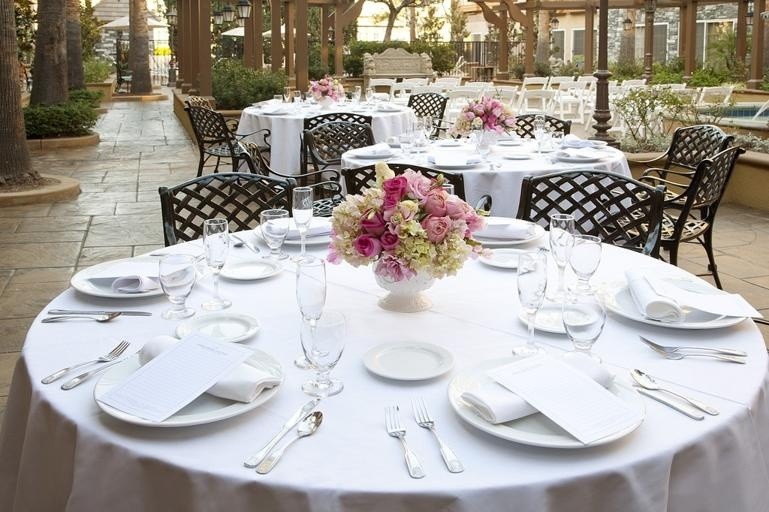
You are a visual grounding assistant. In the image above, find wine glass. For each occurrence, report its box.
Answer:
[299,309,348,396]
[154,251,197,321]
[275,82,374,112]
[517,212,609,359]
[288,186,318,265]
[401,115,545,161]
[203,217,233,309]
[258,209,292,263]
[292,257,327,370]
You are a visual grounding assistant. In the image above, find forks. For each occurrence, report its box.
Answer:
[380,402,427,481]
[636,336,750,370]
[41,340,131,386]
[39,310,125,326]
[410,395,465,476]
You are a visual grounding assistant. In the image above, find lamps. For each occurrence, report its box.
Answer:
[550,11,559,29]
[622,8,633,30]
[167,6,177,26]
[212,0,252,27]
[745,2,753,25]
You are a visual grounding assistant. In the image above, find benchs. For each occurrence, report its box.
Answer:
[362,47,434,93]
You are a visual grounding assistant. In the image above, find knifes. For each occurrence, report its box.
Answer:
[244,397,322,468]
[47,308,152,317]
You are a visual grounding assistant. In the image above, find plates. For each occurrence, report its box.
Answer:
[219,260,282,281]
[478,247,538,270]
[350,137,611,168]
[251,215,334,247]
[263,100,402,113]
[468,216,544,247]
[446,357,649,452]
[175,312,261,347]
[69,255,168,300]
[599,273,748,333]
[93,345,286,427]
[363,339,454,382]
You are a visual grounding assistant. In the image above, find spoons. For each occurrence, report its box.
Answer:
[258,410,329,478]
[628,368,722,420]
